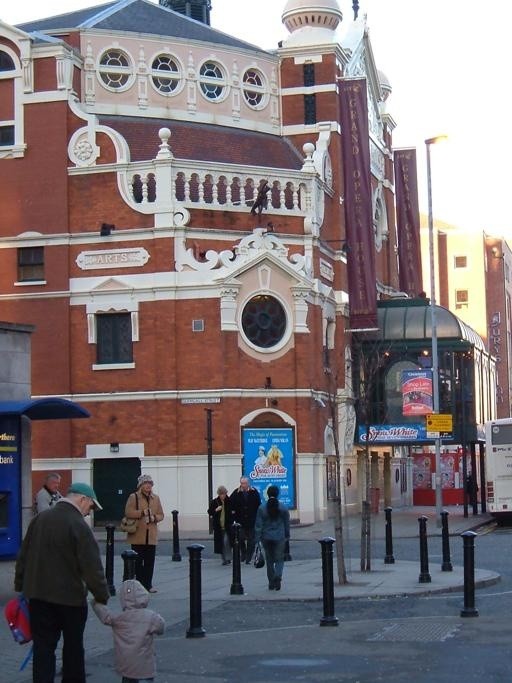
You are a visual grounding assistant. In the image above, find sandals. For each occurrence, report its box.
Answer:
[483,416,512,528]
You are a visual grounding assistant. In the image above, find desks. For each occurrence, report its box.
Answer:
[421,132,450,530]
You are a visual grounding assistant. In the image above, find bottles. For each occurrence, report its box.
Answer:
[137,474,153,488]
[68,483,102,511]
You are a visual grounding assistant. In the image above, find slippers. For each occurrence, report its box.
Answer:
[5,596,32,644]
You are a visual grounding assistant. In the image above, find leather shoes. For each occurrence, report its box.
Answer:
[144,515,155,523]
[143,508,151,516]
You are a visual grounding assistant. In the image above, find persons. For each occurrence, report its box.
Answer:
[228,475,260,563]
[90,577,167,682]
[13,481,111,681]
[207,485,232,565]
[254,444,268,466]
[124,472,164,593]
[254,485,292,590]
[34,470,63,515]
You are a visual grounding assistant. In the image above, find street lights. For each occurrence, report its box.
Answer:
[120,517,139,533]
[254,553,264,568]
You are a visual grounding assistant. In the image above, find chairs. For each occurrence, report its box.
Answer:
[223,560,229,564]
[149,588,156,593]
[241,555,251,563]
[284,555,291,560]
[269,576,281,590]
